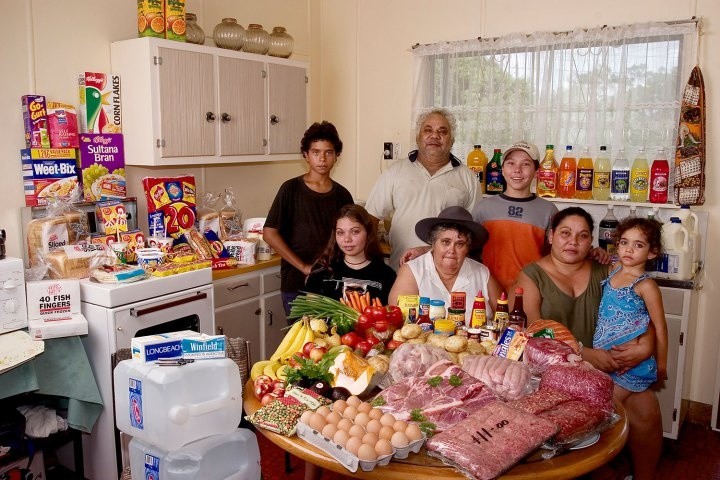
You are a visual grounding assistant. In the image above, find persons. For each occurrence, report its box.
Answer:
[365,107,483,277]
[389,205,506,326]
[400,142,612,295]
[263,120,354,333]
[303,204,398,337]
[516,205,670,480]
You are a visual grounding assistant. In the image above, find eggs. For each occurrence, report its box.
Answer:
[299,396,422,460]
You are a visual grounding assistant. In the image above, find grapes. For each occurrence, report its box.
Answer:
[82,162,126,189]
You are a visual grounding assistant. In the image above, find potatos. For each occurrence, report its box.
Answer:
[366,353,392,374]
[392,324,495,366]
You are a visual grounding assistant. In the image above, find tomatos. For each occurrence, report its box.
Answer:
[341,306,403,355]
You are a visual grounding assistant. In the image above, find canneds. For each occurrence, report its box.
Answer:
[429,300,456,338]
[245,229,272,260]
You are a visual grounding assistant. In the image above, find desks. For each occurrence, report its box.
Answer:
[245,378,629,480]
[0,334,104,480]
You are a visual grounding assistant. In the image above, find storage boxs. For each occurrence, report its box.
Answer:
[131,330,225,363]
[21,72,126,207]
[26,280,88,340]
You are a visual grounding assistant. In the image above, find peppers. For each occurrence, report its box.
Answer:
[384,305,402,327]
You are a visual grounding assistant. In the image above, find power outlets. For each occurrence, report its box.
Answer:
[384,143,402,160]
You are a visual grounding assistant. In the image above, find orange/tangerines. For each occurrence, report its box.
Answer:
[138,13,186,35]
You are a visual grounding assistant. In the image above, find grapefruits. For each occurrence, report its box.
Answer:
[326,352,378,396]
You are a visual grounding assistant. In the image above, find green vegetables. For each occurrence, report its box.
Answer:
[371,376,462,438]
[282,352,337,383]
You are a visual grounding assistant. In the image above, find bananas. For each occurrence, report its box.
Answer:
[270,314,314,363]
[251,360,289,380]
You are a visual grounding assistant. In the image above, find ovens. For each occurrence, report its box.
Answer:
[80,268,217,480]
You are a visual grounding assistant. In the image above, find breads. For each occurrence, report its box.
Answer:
[183,209,259,258]
[25,212,107,281]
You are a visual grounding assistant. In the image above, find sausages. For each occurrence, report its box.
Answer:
[461,356,528,400]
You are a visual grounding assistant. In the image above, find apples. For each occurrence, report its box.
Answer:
[253,376,287,406]
[289,343,328,369]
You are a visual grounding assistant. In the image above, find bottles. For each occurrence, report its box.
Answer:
[577,148,593,200]
[493,292,510,334]
[434,319,455,336]
[485,148,507,195]
[630,148,650,203]
[111,242,127,264]
[184,13,293,58]
[467,145,488,194]
[558,146,576,199]
[609,146,631,201]
[536,144,560,198]
[509,288,528,333]
[468,328,481,343]
[593,146,611,200]
[470,290,487,329]
[429,300,446,322]
[414,297,435,334]
[447,307,466,328]
[598,204,702,280]
[649,146,670,203]
[247,230,271,261]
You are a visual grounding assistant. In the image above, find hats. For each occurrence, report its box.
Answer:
[415,206,490,256]
[501,142,539,171]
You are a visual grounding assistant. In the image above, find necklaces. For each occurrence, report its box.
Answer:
[343,256,368,265]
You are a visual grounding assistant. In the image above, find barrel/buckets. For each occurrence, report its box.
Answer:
[112,354,244,452]
[128,428,261,480]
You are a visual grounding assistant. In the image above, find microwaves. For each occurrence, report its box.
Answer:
[0,256,28,335]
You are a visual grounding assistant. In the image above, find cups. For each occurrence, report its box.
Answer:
[136,248,164,269]
[498,315,525,339]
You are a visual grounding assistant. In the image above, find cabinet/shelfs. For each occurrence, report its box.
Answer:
[214,265,287,367]
[110,37,310,166]
[656,286,699,439]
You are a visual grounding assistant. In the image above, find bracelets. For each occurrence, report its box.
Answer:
[578,342,583,356]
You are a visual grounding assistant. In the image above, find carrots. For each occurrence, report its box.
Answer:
[339,290,382,314]
